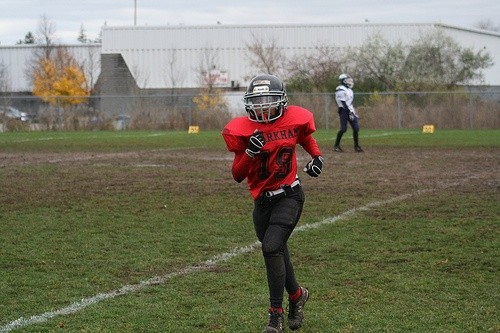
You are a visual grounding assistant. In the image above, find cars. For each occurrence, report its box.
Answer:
[66,106,134,132]
[0,105,29,133]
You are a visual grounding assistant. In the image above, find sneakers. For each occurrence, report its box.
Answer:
[262,306,284,333]
[285,287,309,331]
[333,145,344,153]
[354,145,363,152]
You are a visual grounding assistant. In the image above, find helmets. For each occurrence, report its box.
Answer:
[243,75,288,122]
[338,74,354,88]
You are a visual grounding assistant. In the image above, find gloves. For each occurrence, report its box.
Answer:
[306,155,323,177]
[245,131,265,158]
[348,112,355,122]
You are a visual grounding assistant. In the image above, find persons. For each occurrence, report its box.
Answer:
[222,75,324,333]
[332,73,365,152]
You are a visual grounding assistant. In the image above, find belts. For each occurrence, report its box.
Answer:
[262,174,299,197]
